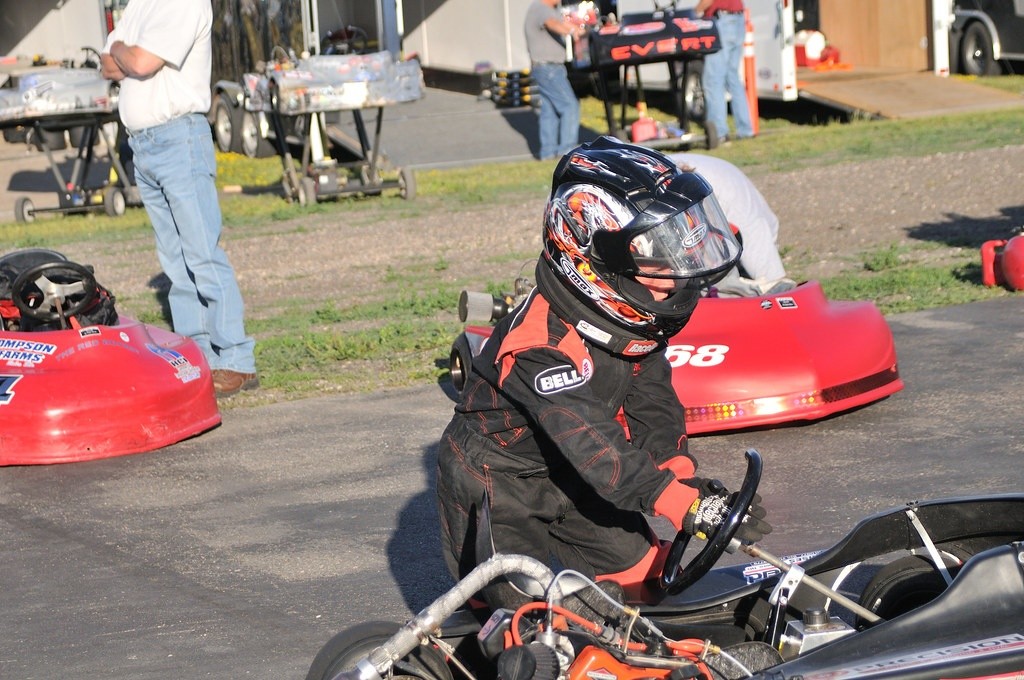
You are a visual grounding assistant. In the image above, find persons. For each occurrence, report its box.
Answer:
[524,0,585,160]
[435,135,772,632]
[693,0,756,143]
[101,0,261,396]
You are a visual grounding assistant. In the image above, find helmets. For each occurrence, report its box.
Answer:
[535,135,741,357]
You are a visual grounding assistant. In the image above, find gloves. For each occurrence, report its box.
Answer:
[677,476,772,541]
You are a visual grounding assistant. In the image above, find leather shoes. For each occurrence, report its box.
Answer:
[211,369,258,398]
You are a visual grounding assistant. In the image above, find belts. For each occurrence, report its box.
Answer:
[716,8,744,16]
